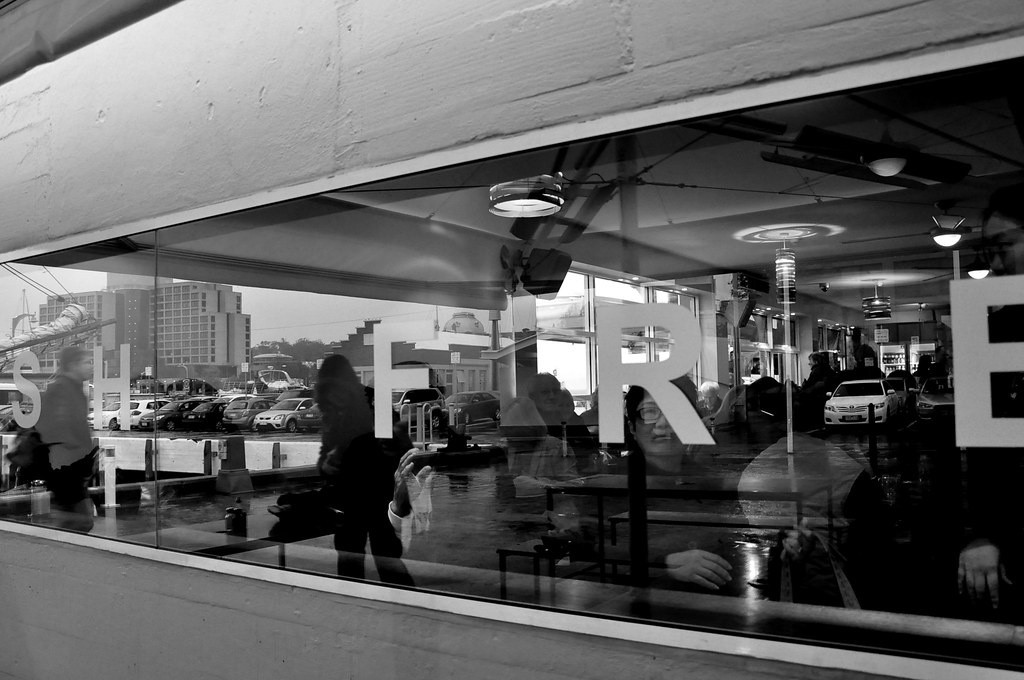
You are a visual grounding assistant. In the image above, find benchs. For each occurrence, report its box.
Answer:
[495,536,703,603]
[607,511,850,590]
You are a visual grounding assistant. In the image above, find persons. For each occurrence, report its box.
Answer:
[800,353,835,390]
[624,376,733,590]
[933,429,1024,624]
[37,346,97,530]
[389,389,516,573]
[316,355,417,586]
[501,374,597,515]
[760,472,891,610]
[699,380,723,416]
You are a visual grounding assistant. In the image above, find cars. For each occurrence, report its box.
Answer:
[824,378,901,432]
[0,369,322,434]
[912,376,955,424]
[442,390,501,426]
[881,377,910,414]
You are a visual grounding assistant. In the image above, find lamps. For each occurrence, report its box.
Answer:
[931,232,962,248]
[861,283,893,320]
[489,175,563,219]
[967,269,990,279]
[864,158,908,177]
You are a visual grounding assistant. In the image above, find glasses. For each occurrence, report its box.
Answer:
[635,407,663,419]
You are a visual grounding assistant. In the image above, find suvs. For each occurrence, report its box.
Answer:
[372,387,446,430]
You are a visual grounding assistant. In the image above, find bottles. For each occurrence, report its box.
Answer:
[224,496,248,537]
[30,480,46,515]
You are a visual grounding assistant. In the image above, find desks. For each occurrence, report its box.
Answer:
[540,473,832,603]
